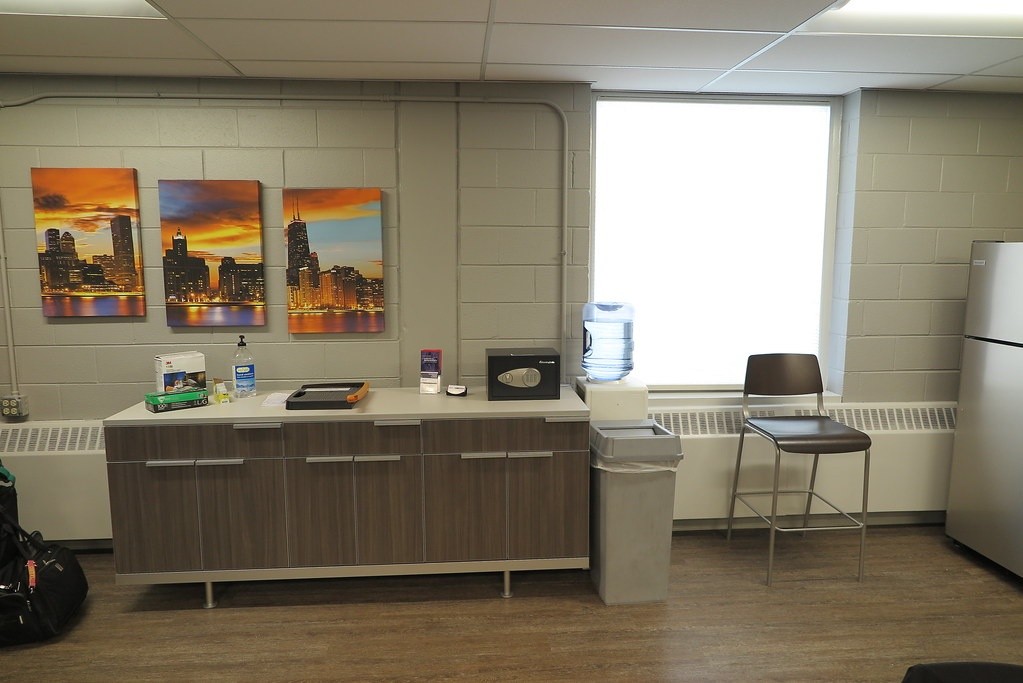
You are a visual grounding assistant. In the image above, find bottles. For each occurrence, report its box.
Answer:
[581,301,635,381]
[231,336,256,398]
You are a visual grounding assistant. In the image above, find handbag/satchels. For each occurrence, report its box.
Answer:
[0,510,89,646]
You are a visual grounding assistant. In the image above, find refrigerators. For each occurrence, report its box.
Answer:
[944,239,1022,584]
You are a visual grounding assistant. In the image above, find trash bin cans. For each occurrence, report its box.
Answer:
[589,420,684,606]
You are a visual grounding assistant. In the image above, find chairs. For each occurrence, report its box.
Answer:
[724,353,872,587]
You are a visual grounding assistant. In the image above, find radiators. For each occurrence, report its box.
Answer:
[0,420,114,550]
[648,401,959,532]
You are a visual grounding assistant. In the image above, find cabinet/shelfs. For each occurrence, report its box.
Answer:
[102,383,591,609]
